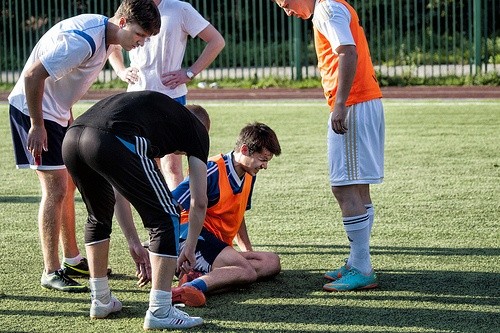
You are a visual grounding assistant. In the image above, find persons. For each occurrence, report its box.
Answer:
[107,0,225,247]
[170,119,281,306]
[62,90,210,330]
[8,0,160,292]
[275,0,385,292]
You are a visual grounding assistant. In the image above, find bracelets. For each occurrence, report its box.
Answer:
[185,68,196,79]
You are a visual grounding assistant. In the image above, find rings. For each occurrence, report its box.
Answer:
[38,145,42,146]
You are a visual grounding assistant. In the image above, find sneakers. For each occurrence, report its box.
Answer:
[90,290,123,318]
[143,305,204,330]
[177,269,202,287]
[324,262,352,281]
[323,265,378,291]
[171,282,206,307]
[41,268,88,292]
[61,258,112,278]
